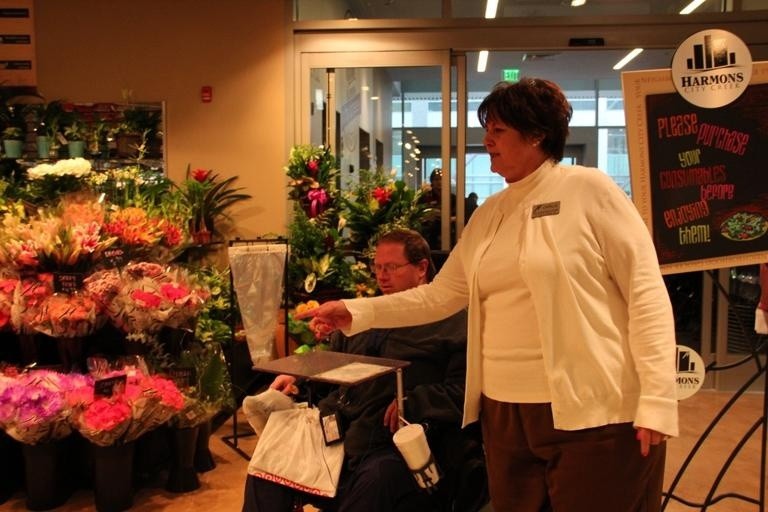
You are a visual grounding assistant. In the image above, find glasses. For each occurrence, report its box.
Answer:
[369,258,421,275]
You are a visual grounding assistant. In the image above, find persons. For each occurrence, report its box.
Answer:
[292,76,680,512]
[465,192,479,225]
[416,167,456,250]
[239,226,489,511]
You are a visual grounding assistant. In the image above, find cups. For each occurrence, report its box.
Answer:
[392,424,440,489]
